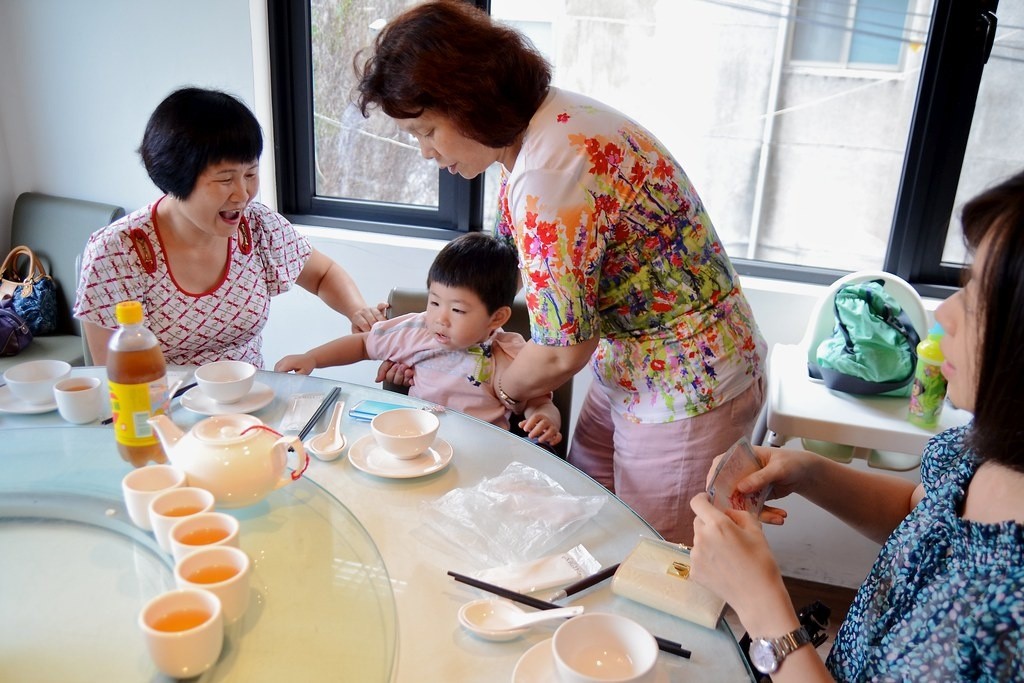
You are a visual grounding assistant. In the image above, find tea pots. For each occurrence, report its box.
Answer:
[147,415,308,509]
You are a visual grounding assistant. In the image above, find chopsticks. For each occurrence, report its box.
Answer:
[101,382,197,425]
[447,571,691,659]
[287,387,341,452]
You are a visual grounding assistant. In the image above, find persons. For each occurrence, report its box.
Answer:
[689,170,1024,683]
[277,232,562,445]
[73,89,390,371]
[360,0,768,547]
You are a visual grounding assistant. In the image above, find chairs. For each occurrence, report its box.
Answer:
[0,190,126,376]
[383,286,571,466]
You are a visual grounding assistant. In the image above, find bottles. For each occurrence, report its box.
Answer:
[107,301,170,468]
[907,322,947,430]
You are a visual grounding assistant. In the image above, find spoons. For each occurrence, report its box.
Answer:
[462,601,584,631]
[312,400,345,451]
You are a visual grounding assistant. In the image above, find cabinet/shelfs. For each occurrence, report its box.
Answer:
[749,345,976,588]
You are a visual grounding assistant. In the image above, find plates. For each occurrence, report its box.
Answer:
[0,371,57,414]
[180,382,275,415]
[511,637,670,683]
[348,434,453,478]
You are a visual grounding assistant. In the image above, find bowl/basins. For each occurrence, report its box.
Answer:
[551,613,659,683]
[3,359,72,404]
[458,602,530,641]
[303,432,346,461]
[371,409,439,460]
[195,361,256,404]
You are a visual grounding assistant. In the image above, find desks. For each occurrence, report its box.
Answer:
[0,366,757,683]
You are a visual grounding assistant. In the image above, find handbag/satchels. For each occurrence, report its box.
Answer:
[0,246,58,334]
[0,305,33,357]
[819,282,920,397]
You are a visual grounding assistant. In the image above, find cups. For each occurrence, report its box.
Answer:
[122,465,187,531]
[173,546,250,625]
[148,488,216,554]
[54,376,101,424]
[170,512,239,562]
[138,589,223,678]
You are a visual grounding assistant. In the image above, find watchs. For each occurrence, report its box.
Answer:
[498,376,517,406]
[749,626,811,674]
